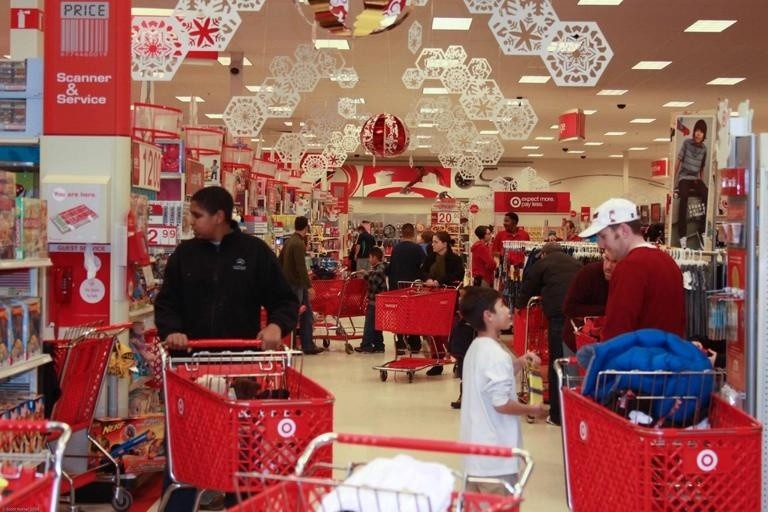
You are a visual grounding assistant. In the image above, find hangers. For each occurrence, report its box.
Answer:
[501,240,704,266]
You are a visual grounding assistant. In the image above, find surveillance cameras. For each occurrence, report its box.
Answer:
[562,148,569,153]
[617,103,626,110]
[581,156,586,159]
[229,62,241,75]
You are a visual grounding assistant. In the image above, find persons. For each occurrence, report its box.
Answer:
[458,287,550,511]
[277,217,325,356]
[560,245,618,388]
[352,224,466,375]
[676,120,708,250]
[493,213,530,269]
[576,197,686,340]
[470,226,497,287]
[513,243,585,426]
[545,220,582,242]
[156,185,300,511]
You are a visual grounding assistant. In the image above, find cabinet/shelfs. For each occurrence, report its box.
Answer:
[255,213,469,281]
[0,257,54,469]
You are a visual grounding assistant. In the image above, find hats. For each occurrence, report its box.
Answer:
[577,198,640,238]
[535,242,562,258]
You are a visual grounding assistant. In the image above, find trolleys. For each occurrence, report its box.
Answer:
[375,278,464,383]
[513,295,548,405]
[43,318,136,511]
[156,337,335,511]
[569,315,601,385]
[1,418,73,511]
[219,431,534,511]
[308,264,371,355]
[553,350,763,510]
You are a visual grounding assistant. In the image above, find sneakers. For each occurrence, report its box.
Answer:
[427,366,443,376]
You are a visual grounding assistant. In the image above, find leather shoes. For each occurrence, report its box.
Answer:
[311,346,424,355]
